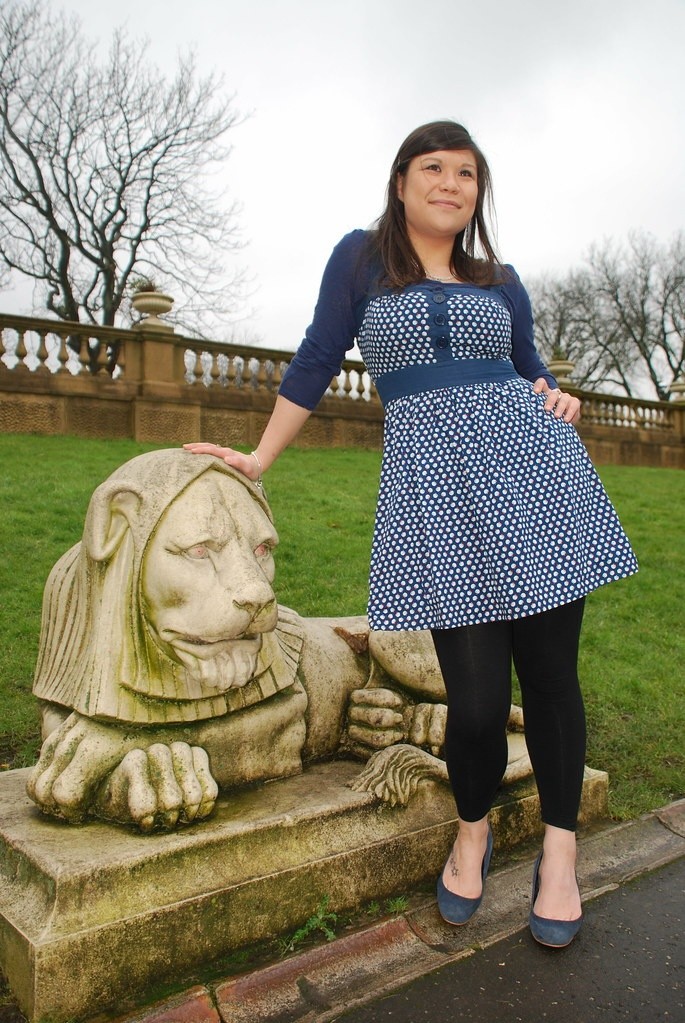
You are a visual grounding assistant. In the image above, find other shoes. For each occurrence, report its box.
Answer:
[528,848,584,947]
[437,824,493,926]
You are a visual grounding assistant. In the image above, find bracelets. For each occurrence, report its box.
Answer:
[251,452,263,489]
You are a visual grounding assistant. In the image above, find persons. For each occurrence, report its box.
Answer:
[183,120,639,947]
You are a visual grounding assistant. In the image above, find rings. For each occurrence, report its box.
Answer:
[216,444,222,449]
[552,388,562,399]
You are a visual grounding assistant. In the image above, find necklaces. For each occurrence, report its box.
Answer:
[415,259,457,279]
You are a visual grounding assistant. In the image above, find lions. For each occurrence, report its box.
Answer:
[25,448,534,835]
